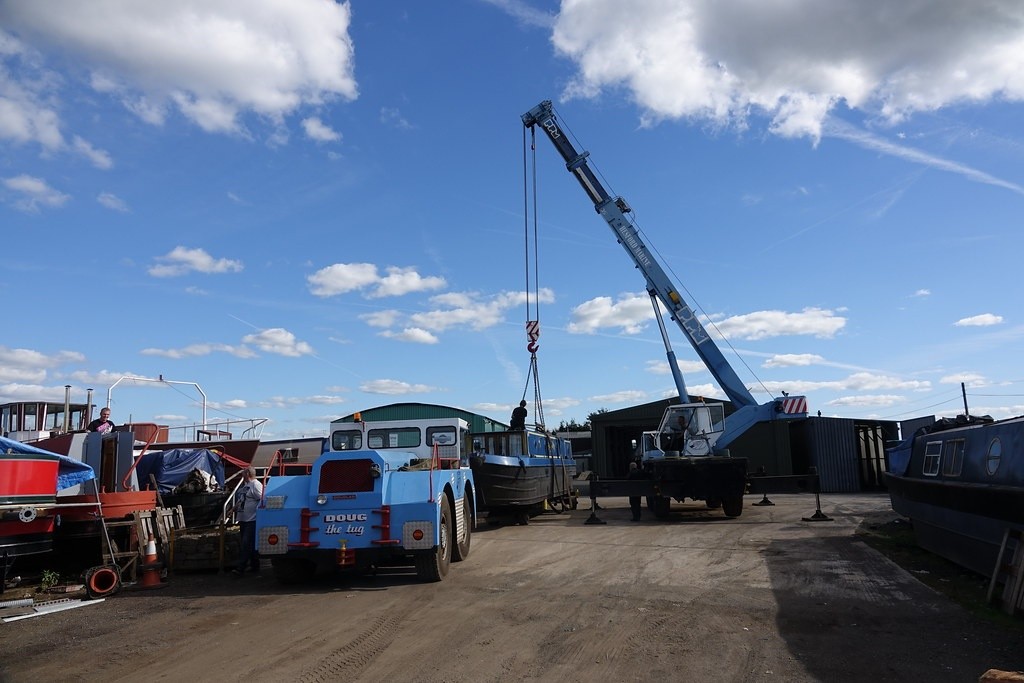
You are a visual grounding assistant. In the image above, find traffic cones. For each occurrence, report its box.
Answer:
[130,533,170,590]
[85,563,123,601]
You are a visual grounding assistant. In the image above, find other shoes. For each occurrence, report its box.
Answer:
[631,518,640,521]
[233,567,244,574]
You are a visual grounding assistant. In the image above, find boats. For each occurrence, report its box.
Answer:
[0,377,206,575]
[470,429,577,506]
[132,446,231,527]
[881,415,1024,585]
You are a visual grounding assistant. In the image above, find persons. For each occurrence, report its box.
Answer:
[227,466,264,575]
[510,400,527,430]
[86,407,116,435]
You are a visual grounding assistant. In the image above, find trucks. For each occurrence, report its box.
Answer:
[254,412,479,592]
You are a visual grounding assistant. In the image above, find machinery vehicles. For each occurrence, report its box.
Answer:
[519,98,836,526]
